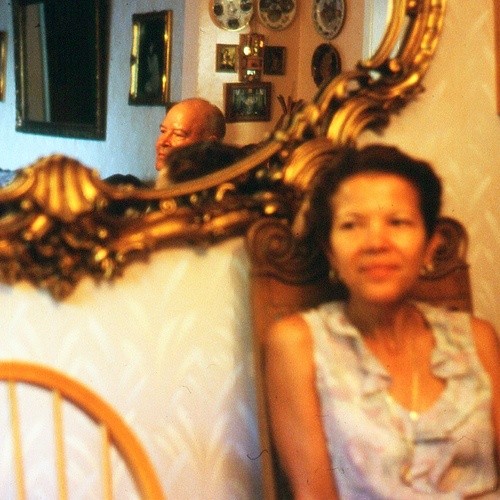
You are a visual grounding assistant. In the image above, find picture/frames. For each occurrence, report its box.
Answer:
[128,9,172,107]
[263,45,287,75]
[223,82,272,122]
[215,44,242,73]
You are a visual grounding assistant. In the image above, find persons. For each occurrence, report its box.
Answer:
[151,98,225,172]
[153,140,258,191]
[264,143,499,500]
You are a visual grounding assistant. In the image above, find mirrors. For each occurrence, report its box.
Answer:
[0,1,449,302]
[10,0,112,141]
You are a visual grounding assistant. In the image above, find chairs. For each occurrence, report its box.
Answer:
[2,360,164,498]
[242,185,475,499]
